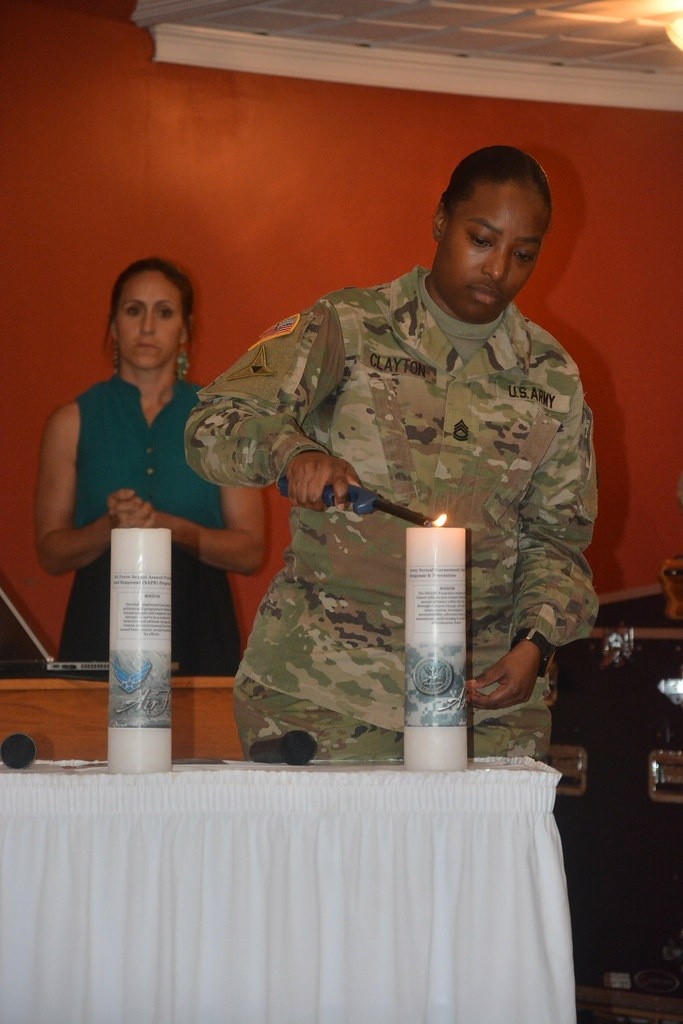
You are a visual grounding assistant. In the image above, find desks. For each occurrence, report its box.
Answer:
[0,675,240,766]
[3,752,579,1022]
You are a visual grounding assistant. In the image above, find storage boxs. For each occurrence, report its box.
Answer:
[543,580,683,994]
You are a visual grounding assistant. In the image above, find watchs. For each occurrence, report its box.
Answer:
[509,625,559,679]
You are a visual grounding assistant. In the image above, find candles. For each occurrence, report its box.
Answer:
[403,513,473,770]
[103,525,176,773]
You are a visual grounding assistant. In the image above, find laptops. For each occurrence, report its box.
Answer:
[1,589,180,674]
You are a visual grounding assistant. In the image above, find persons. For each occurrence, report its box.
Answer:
[30,257,269,677]
[183,142,598,761]
